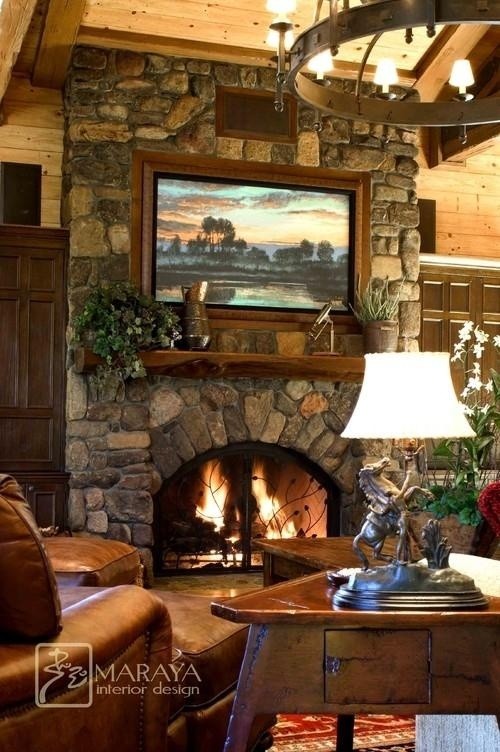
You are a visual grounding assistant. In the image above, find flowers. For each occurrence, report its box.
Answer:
[421,320,500,527]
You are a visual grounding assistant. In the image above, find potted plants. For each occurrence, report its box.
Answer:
[71,276,183,403]
[348,268,414,353]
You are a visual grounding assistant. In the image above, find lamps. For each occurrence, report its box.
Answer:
[334,350,487,610]
[265,0,500,141]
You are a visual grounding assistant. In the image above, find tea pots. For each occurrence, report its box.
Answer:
[180,280,214,352]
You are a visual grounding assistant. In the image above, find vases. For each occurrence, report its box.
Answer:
[368,505,496,563]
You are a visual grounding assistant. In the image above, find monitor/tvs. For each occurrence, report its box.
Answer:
[141,161,363,324]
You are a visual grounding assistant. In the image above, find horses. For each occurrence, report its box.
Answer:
[352,456,431,571]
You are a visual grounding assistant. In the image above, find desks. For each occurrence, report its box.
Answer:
[210,532,498,752]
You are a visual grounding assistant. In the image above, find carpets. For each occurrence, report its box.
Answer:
[265,712,417,752]
